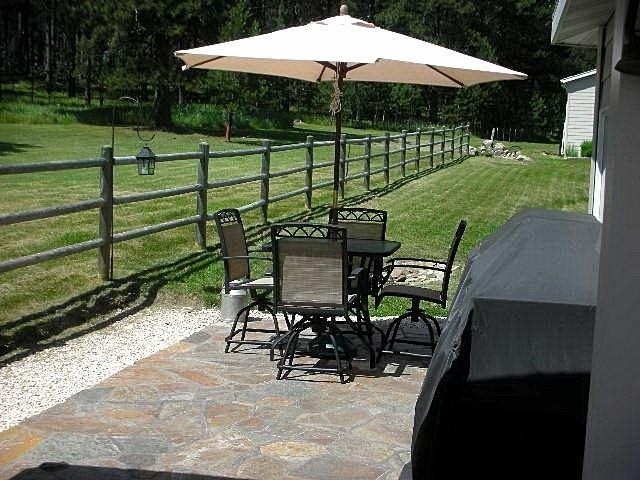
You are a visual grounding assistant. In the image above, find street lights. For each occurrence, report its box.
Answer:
[107,94,157,279]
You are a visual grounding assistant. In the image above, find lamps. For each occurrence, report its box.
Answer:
[137,141,156,174]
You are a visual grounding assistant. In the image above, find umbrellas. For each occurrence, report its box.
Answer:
[173,4,528,241]
